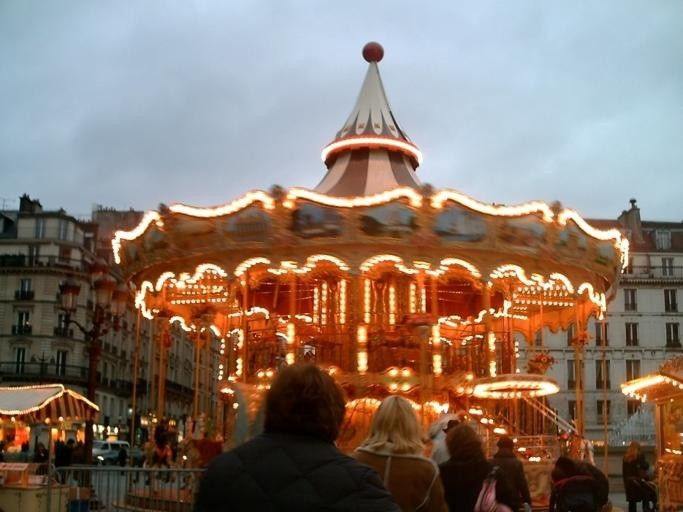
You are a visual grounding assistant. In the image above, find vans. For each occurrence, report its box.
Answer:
[92,440,131,464]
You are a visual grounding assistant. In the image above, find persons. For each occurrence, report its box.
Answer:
[118,444,127,475]
[431,419,532,512]
[192,363,393,512]
[622,440,656,511]
[146,432,172,468]
[63,437,77,457]
[350,395,448,512]
[546,456,609,512]
[33,442,48,473]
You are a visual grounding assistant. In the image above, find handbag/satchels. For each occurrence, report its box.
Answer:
[474,478,512,512]
